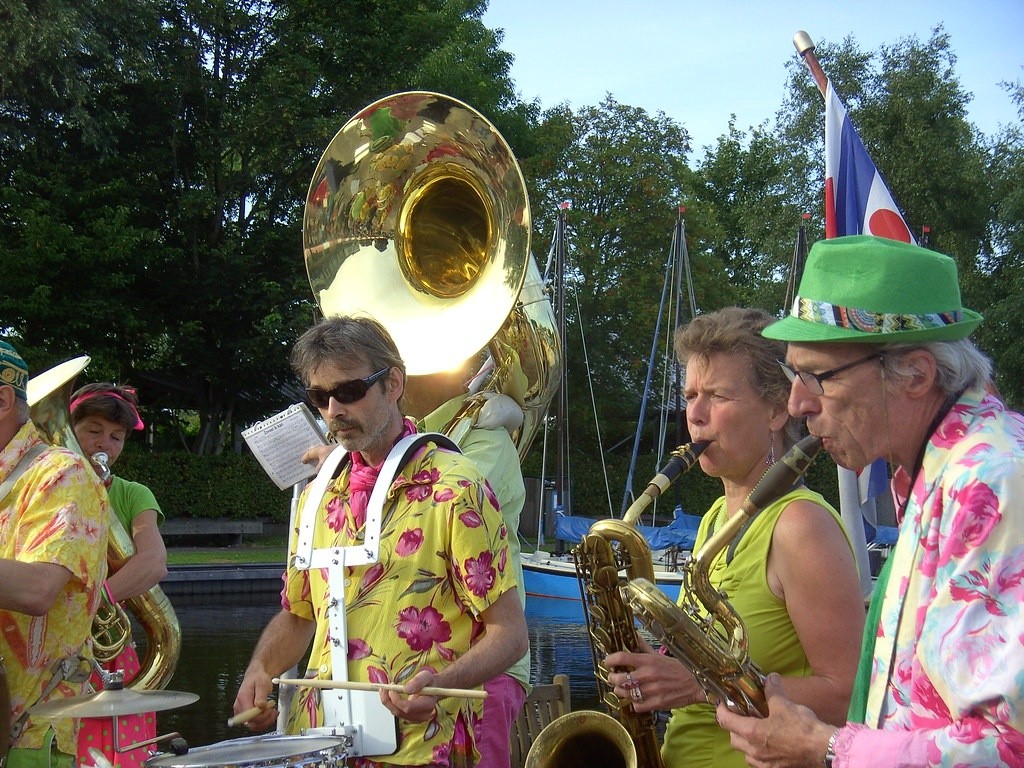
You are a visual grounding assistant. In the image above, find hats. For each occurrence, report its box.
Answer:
[0,340,29,401]
[760,234,986,343]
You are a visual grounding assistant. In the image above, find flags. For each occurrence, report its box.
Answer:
[825,78,917,601]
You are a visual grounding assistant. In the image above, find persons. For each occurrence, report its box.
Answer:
[312,93,523,235]
[234,315,529,768]
[68,381,168,768]
[0,337,110,768]
[604,236,1024,768]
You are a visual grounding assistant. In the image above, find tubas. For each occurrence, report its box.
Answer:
[23,354,183,692]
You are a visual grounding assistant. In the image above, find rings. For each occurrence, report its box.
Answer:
[629,690,642,701]
[620,672,642,688]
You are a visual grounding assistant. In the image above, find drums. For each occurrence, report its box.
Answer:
[143,733,351,768]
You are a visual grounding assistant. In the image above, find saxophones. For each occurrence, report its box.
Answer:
[616,433,826,719]
[521,438,717,768]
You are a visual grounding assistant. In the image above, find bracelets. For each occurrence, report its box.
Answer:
[98,581,114,611]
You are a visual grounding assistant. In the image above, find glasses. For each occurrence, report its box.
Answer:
[775,348,882,396]
[305,367,389,408]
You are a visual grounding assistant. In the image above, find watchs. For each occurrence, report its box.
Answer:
[825,728,839,768]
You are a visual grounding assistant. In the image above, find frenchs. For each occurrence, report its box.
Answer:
[300,87,565,468]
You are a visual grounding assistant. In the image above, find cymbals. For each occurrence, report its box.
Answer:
[24,686,200,721]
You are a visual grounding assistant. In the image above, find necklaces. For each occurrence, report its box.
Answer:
[715,461,779,530]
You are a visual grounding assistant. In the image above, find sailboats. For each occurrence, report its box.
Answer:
[516,200,901,615]
